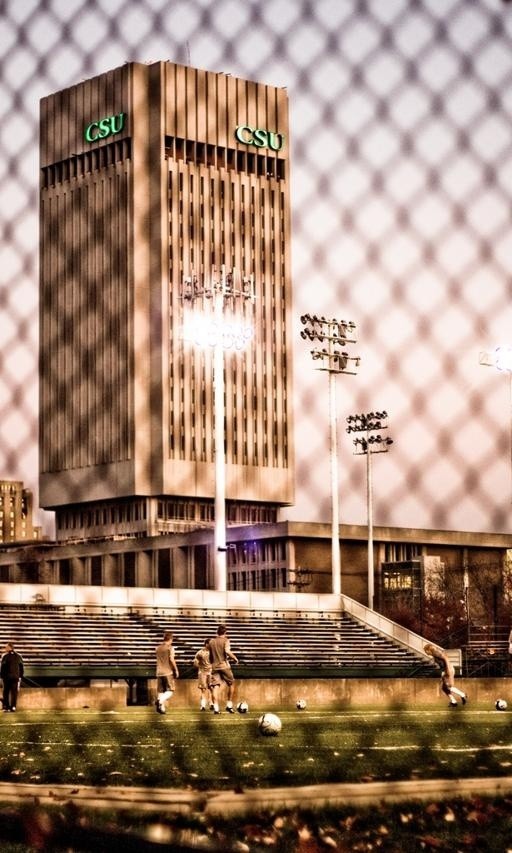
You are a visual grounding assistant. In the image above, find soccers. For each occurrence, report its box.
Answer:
[495,698,507,711]
[236,702,248,713]
[296,700,306,710]
[258,713,281,736]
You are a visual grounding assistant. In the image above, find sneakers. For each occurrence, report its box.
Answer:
[448,691,467,707]
[200,705,234,715]
[156,698,165,713]
[4,706,16,712]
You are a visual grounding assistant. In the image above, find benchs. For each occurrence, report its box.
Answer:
[0,607,437,670]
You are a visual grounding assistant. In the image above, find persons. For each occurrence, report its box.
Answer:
[154,633,180,714]
[192,638,214,711]
[208,625,239,714]
[0,643,26,713]
[423,644,468,708]
